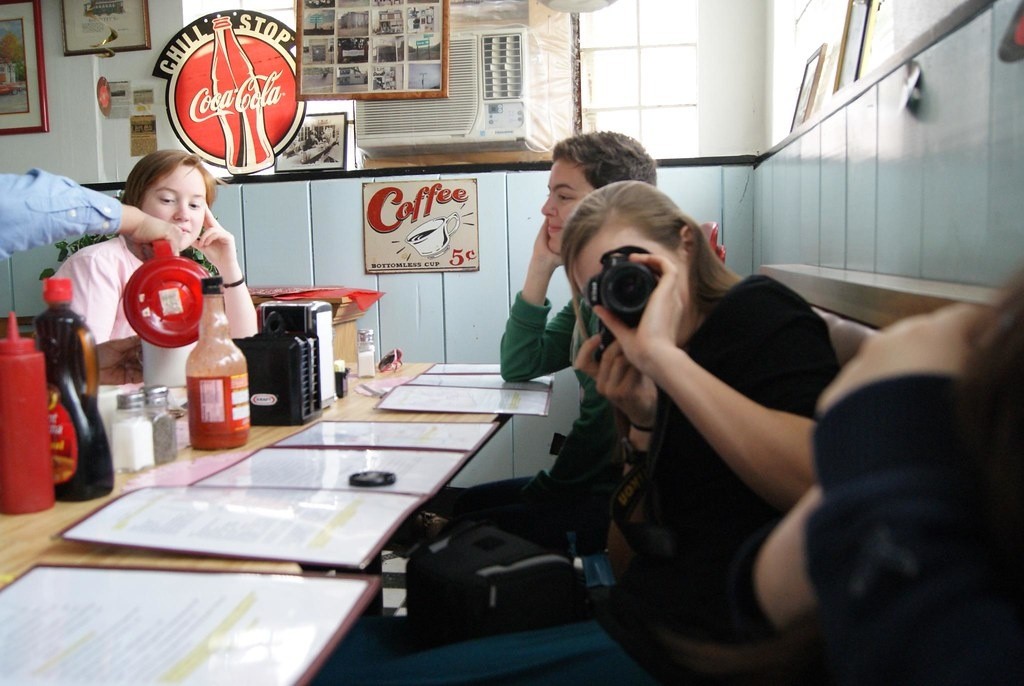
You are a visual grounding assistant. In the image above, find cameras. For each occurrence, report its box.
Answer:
[586,255,660,361]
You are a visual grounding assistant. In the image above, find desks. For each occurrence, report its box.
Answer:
[0,362,513,686]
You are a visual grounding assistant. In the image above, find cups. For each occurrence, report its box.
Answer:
[139,337,199,386]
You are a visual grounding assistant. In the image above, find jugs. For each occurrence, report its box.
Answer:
[123,239,213,349]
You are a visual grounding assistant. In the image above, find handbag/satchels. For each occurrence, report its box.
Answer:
[405,521,584,643]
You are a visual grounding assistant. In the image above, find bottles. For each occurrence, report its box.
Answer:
[141,386,178,463]
[26,279,115,500]
[110,391,156,472]
[356,329,376,378]
[0,308,56,515]
[182,275,249,450]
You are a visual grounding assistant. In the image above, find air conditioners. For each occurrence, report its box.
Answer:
[351,24,533,157]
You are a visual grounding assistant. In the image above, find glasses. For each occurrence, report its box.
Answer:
[379,348,403,372]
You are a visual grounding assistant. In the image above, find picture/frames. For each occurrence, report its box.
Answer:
[789,44,828,133]
[295,0,450,102]
[60,0,152,56]
[0,0,49,135]
[274,111,348,175]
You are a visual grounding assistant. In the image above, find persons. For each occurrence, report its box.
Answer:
[310,130,1024,686]
[1,167,181,388]
[49,149,258,345]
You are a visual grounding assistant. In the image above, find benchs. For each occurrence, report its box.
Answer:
[751,262,1004,373]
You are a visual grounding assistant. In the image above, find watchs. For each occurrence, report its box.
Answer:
[620,437,648,466]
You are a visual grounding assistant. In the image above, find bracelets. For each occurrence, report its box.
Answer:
[223,277,244,288]
[632,422,654,432]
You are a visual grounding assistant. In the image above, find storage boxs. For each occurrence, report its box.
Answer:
[249,296,368,366]
